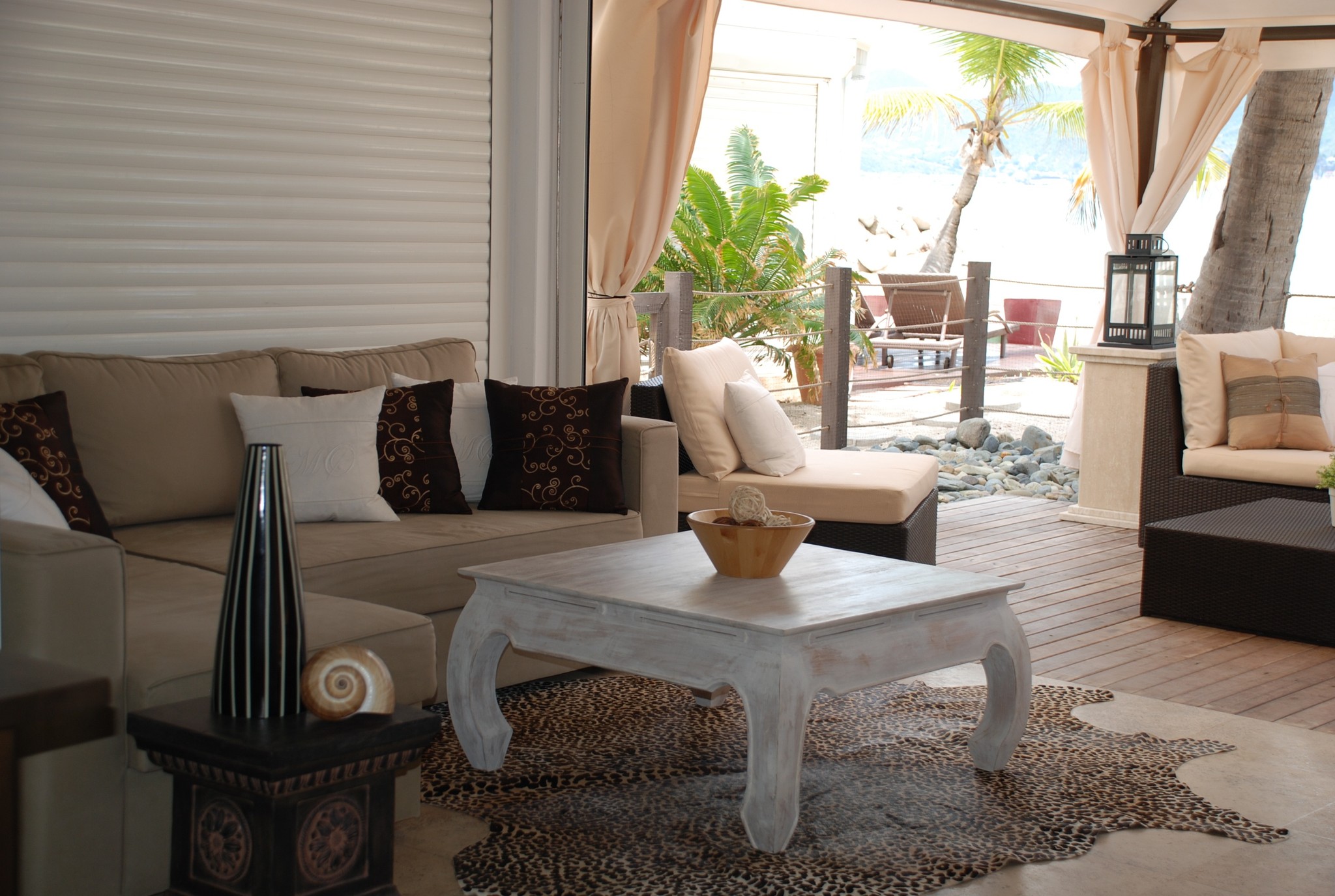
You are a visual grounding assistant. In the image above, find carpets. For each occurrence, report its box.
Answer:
[411,668,1291,896]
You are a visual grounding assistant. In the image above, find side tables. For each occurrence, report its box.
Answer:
[1056,343,1180,530]
[124,696,444,896]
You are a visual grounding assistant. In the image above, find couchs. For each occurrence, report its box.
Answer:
[0,336,678,896]
[629,337,938,569]
[1136,325,1335,548]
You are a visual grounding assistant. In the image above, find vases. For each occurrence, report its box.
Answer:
[205,443,310,725]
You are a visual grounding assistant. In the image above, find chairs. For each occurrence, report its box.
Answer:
[862,273,1020,371]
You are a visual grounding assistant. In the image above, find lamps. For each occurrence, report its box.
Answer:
[1097,233,1179,351]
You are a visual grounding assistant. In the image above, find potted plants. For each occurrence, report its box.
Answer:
[724,125,880,409]
[1314,450,1335,528]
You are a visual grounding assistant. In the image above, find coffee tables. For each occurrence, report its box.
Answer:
[1136,496,1335,650]
[443,526,1035,858]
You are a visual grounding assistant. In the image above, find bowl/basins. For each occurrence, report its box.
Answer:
[686,509,815,579]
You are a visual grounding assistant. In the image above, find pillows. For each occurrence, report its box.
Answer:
[229,384,404,529]
[301,378,473,517]
[661,337,762,482]
[476,377,631,517]
[1219,351,1335,452]
[1275,328,1335,367]
[389,372,520,503]
[0,390,123,545]
[722,367,808,479]
[0,448,72,530]
[1317,360,1335,448]
[1175,326,1283,450]
